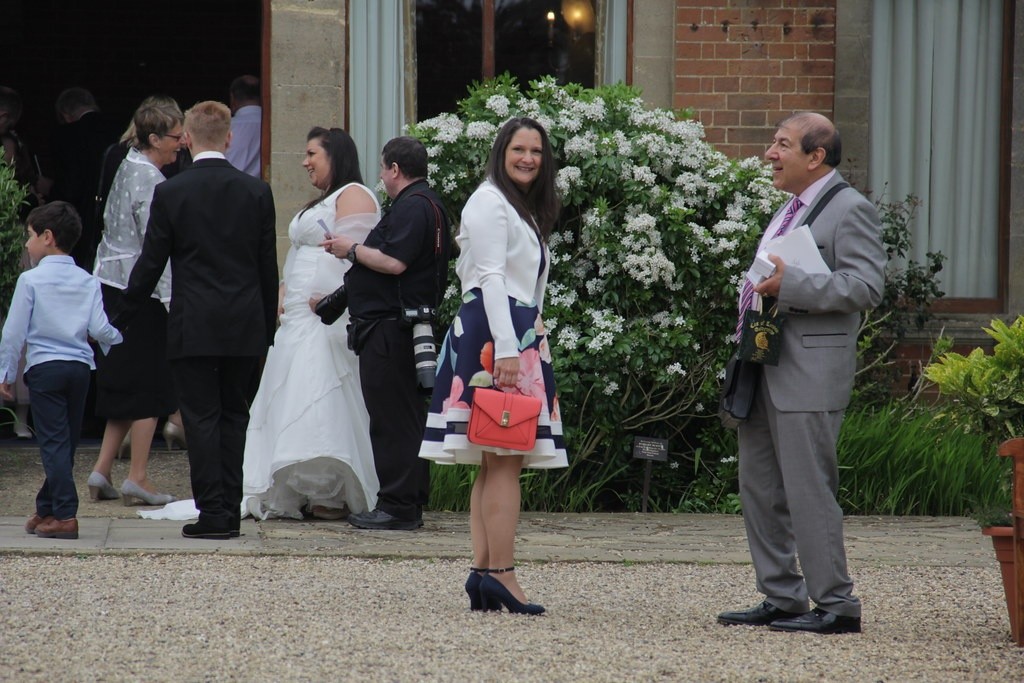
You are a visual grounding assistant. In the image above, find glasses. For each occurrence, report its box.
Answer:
[164,134,183,142]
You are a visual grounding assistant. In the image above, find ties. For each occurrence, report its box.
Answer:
[735,199,804,344]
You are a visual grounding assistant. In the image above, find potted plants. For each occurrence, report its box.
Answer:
[969,505,1017,642]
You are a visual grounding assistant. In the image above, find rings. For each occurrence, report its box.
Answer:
[761,292,769,297]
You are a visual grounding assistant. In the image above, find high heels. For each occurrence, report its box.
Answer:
[120,478,177,506]
[117,432,131,459]
[87,472,121,500]
[163,421,187,451]
[465,566,545,614]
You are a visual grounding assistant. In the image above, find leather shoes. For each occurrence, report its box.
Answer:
[25,513,78,539]
[347,509,424,530]
[717,600,810,627]
[769,606,861,635]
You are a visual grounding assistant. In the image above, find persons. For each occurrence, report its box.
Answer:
[315,135,452,531]
[88,94,187,506]
[0,198,122,539]
[0,75,260,193]
[119,100,279,538]
[713,109,889,636]
[137,125,382,521]
[418,118,569,615]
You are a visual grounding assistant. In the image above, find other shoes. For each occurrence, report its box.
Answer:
[182,518,241,540]
[314,504,350,519]
[13,428,32,438]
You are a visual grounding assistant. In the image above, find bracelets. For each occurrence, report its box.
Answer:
[346,243,358,263]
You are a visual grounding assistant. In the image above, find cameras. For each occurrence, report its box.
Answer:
[314,284,349,325]
[403,304,437,388]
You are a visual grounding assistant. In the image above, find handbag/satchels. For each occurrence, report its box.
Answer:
[86,246,96,273]
[718,353,761,430]
[740,291,787,368]
[468,383,542,452]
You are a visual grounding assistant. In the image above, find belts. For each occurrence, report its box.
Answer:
[356,315,397,322]
